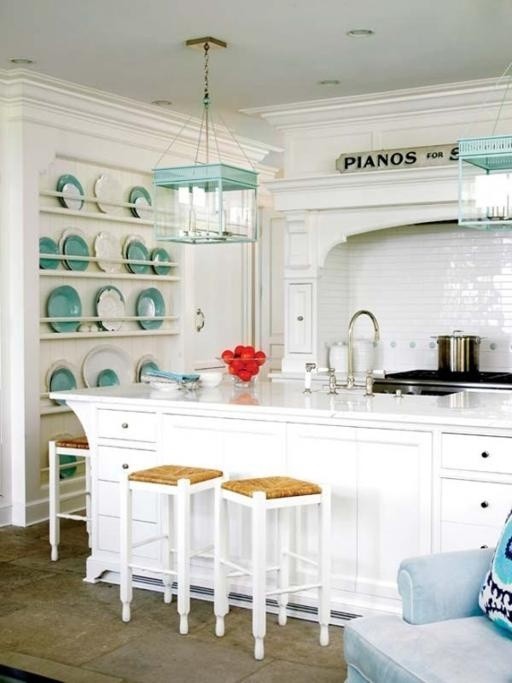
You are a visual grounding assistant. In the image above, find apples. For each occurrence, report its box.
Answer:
[222,345,266,381]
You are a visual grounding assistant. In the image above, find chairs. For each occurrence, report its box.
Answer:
[342,547,510,681]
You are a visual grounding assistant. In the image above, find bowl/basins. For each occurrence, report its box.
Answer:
[216,357,266,386]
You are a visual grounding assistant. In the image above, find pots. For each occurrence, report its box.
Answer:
[437,329,487,374]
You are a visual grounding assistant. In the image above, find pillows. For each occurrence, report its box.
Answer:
[477,506,512,635]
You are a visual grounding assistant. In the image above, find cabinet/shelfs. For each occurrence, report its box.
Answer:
[9,143,190,526]
[180,228,255,371]
[281,276,320,376]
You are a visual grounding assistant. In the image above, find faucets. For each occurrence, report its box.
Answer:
[347,310,380,390]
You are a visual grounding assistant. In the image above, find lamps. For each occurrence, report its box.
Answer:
[456,61,511,232]
[151,34,259,245]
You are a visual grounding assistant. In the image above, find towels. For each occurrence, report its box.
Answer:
[140,374,176,383]
[147,368,201,383]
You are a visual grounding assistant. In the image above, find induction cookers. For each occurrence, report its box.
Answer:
[373,369,512,395]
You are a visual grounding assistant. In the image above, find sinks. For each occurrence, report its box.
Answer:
[370,380,463,398]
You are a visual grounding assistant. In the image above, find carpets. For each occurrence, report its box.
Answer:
[1,663,66,683]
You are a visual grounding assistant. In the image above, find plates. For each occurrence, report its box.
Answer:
[94,285,127,331]
[93,173,121,214]
[96,288,125,330]
[137,356,160,381]
[139,361,158,382]
[59,454,77,477]
[81,345,133,386]
[59,228,91,270]
[129,186,151,218]
[123,233,149,273]
[47,363,76,390]
[151,247,172,274]
[134,195,150,216]
[126,241,149,273]
[94,232,124,271]
[63,235,90,270]
[136,286,165,328]
[49,370,76,404]
[62,182,81,209]
[38,236,59,268]
[97,368,119,386]
[57,172,84,209]
[46,285,82,331]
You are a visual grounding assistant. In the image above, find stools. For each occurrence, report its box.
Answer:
[223,476,331,662]
[121,465,228,638]
